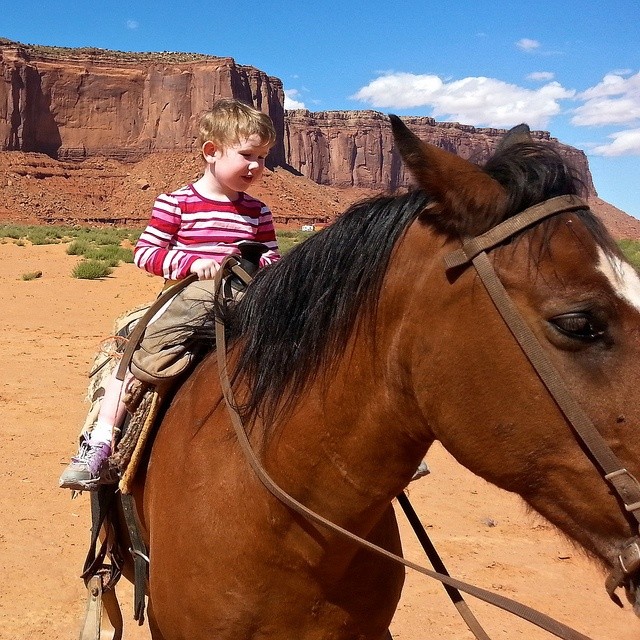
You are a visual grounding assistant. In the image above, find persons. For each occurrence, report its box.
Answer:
[60,97,282,495]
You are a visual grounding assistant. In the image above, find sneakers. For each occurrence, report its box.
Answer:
[59,441,109,489]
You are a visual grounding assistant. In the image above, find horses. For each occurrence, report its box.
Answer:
[90,112,639,639]
